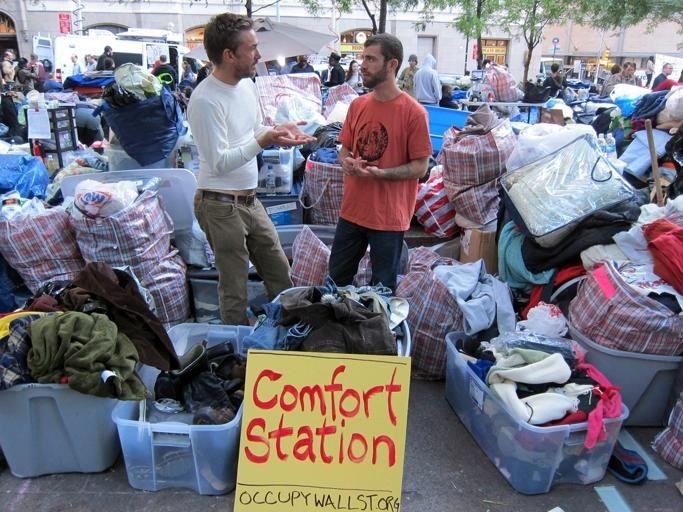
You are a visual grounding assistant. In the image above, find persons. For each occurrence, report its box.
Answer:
[152,56,215,112]
[0,49,47,94]
[185,11,318,325]
[537,56,674,97]
[324,32,434,299]
[70,46,116,72]
[287,49,460,110]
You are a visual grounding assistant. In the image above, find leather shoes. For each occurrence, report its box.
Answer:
[154,339,246,423]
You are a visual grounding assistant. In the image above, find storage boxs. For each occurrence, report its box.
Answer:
[0,76,682,492]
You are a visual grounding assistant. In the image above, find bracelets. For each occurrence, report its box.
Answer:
[340,151,354,167]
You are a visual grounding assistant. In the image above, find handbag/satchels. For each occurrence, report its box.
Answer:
[652,392,683,468]
[289,225,461,383]
[567,257,683,356]
[482,64,525,102]
[414,117,636,246]
[92,75,187,165]
[254,72,360,226]
[0,179,193,330]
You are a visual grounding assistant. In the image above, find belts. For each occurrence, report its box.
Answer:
[204,190,257,206]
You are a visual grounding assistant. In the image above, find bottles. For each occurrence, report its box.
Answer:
[266,164,276,195]
[47,155,55,174]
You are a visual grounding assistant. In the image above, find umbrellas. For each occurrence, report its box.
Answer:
[183,15,338,65]
